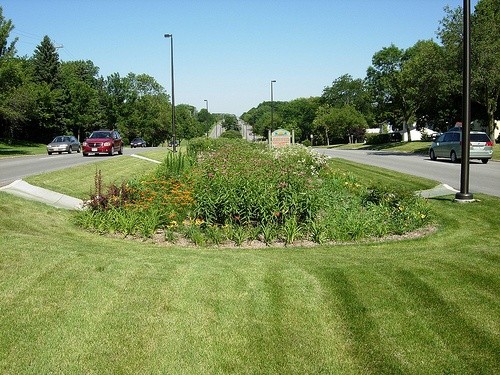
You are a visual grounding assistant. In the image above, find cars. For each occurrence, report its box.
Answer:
[169,141,177,147]
[131,138,146,148]
[47,136,81,155]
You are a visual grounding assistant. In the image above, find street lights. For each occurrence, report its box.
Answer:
[204,100,208,149]
[271,80,276,132]
[164,34,176,158]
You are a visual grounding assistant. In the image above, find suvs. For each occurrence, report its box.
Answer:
[428,131,494,164]
[82,131,123,156]
